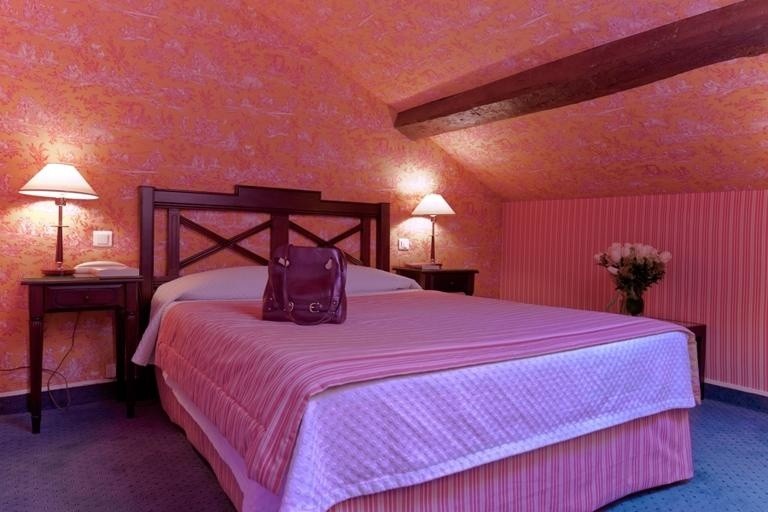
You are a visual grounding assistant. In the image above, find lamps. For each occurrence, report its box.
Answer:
[18,161,100,279]
[411,194,457,266]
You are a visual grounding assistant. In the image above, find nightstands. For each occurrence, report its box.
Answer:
[19,274,145,435]
[392,267,479,296]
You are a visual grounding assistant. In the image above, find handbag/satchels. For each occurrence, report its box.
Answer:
[262,244,347,325]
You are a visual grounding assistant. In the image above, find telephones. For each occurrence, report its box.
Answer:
[72,261,127,274]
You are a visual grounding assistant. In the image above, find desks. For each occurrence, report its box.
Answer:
[669,319,706,401]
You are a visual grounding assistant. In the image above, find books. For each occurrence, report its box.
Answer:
[404,263,442,270]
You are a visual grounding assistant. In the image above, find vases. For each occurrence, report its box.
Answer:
[626,292,642,316]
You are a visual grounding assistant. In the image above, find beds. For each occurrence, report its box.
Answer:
[136,184,701,512]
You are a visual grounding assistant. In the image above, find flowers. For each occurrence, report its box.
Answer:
[593,241,672,313]
